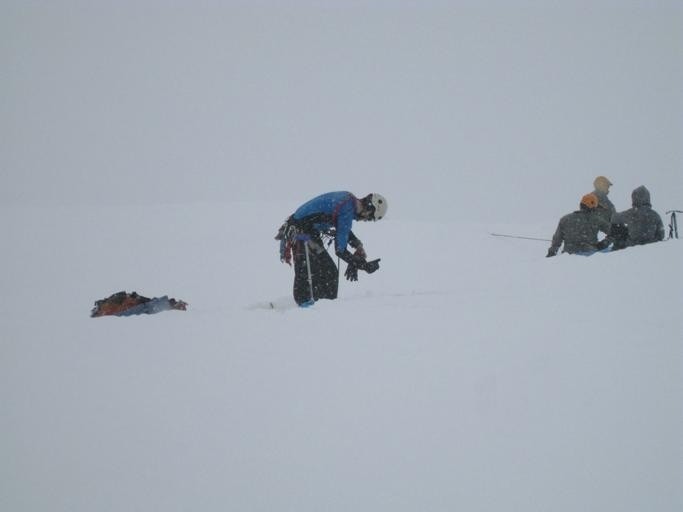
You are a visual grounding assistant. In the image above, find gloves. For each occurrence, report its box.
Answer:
[366,259,380,273]
[344,264,358,282]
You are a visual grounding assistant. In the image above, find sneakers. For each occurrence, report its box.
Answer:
[299,300,314,308]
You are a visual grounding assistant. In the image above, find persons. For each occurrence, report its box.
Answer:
[589,177,616,221]
[274,190,388,307]
[546,192,614,256]
[613,184,665,253]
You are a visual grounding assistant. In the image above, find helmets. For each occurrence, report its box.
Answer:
[371,193,387,222]
[593,176,612,194]
[580,194,599,213]
